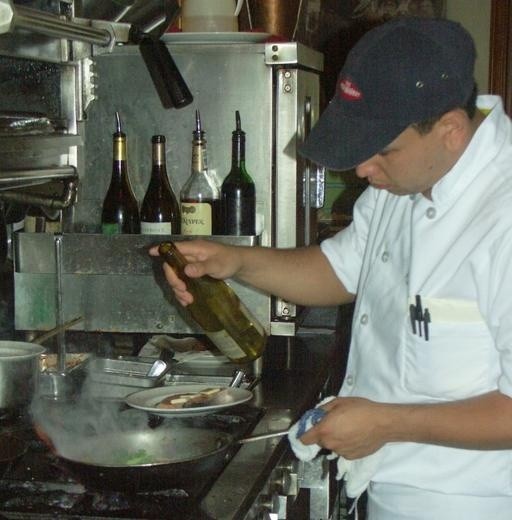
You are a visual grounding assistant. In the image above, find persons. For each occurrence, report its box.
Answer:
[147,18,512,519]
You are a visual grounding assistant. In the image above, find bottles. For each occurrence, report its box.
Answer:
[222,128,253,238]
[139,136,179,234]
[182,129,222,234]
[100,132,141,235]
[159,240,267,363]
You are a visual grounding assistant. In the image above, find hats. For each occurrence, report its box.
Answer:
[294,17,479,175]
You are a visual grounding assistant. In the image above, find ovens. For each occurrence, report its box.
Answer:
[91,41,325,352]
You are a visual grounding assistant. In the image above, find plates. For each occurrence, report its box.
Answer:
[127,383,253,418]
[163,31,272,41]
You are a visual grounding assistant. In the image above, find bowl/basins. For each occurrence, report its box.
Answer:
[1,340,46,410]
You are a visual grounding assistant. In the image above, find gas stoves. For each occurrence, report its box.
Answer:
[3,410,306,520]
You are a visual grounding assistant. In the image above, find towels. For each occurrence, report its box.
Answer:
[287,394,376,500]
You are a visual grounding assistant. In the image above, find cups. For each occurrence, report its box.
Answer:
[181,0,244,33]
[246,0,302,41]
[60,17,131,43]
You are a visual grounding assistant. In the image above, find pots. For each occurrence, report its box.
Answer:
[58,424,290,488]
